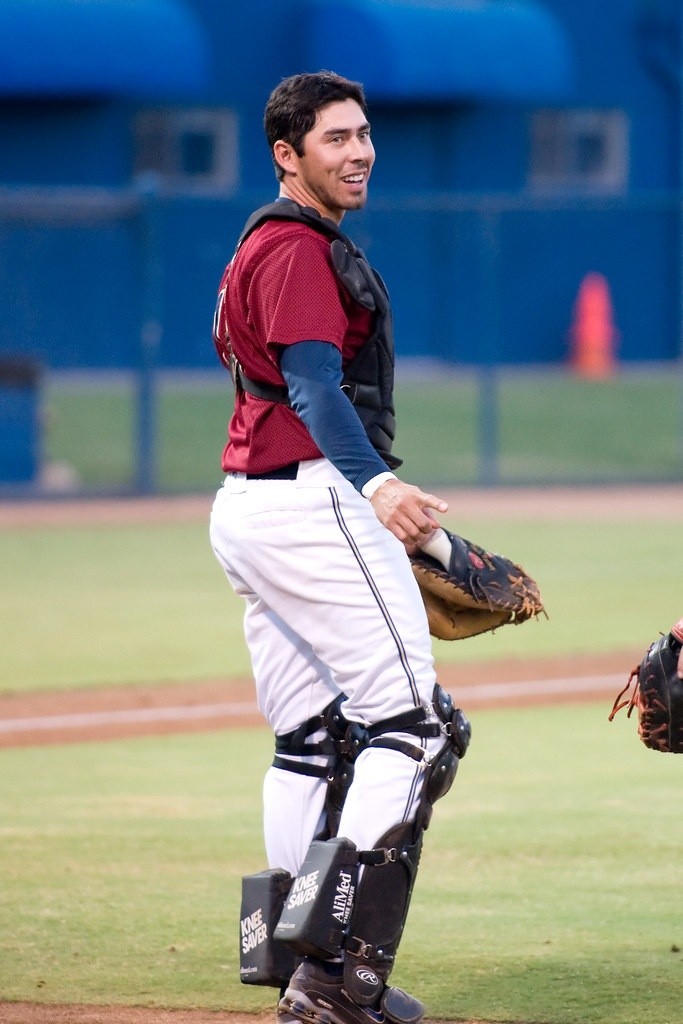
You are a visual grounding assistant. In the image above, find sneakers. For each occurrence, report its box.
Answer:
[278,953,390,1023]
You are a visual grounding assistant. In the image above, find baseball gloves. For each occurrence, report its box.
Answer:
[629,627,683,762]
[400,519,553,650]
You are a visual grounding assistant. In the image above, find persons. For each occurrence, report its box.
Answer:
[206,72,471,1024]
[678,647,683,681]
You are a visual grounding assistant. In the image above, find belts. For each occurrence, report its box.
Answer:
[228,459,302,479]
